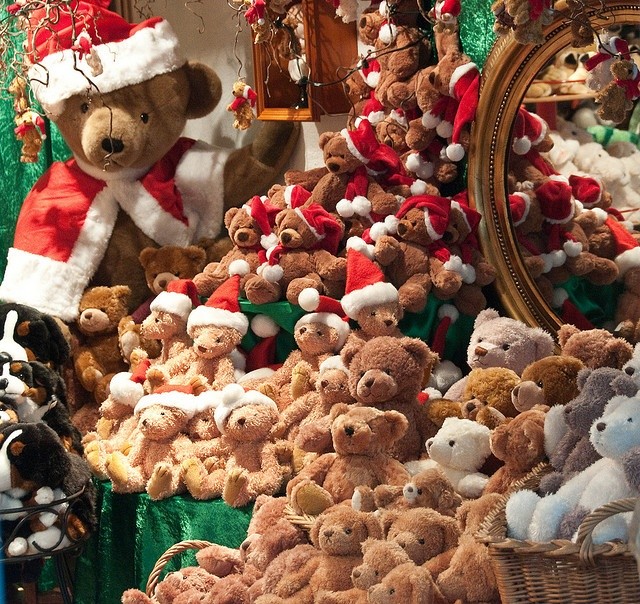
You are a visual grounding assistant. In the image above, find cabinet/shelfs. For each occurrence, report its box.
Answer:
[247,0,357,123]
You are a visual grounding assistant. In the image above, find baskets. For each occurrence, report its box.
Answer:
[484,462,640,604]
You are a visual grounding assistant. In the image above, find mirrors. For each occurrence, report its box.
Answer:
[468,0,640,357]
